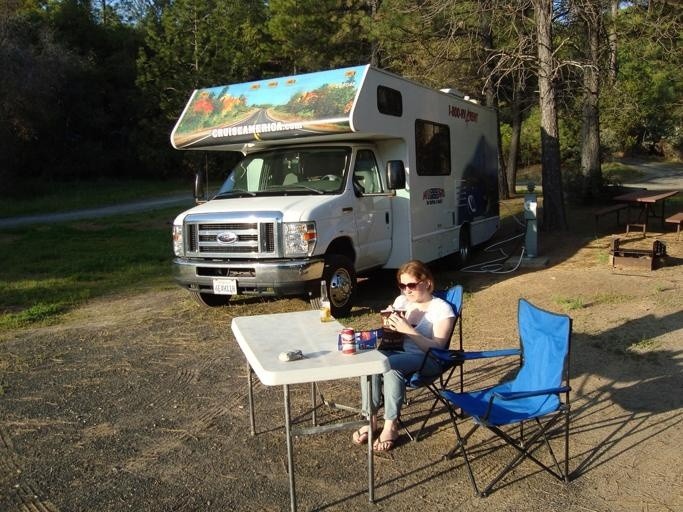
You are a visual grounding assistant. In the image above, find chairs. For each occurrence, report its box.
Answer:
[399,284,573,499]
[279,170,374,192]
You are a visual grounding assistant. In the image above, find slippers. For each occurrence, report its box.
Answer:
[352,430,400,453]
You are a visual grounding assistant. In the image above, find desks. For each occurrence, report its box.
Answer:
[612,189,678,228]
[231,309,390,512]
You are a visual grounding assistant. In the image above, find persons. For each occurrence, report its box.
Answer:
[352,261,455,452]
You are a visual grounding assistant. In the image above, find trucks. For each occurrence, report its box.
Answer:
[168,63,500,319]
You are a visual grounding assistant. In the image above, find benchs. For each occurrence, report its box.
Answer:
[587,205,683,234]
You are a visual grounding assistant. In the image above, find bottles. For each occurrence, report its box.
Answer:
[320,280,331,322]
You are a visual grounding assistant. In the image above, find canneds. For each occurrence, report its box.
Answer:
[341,328,356,355]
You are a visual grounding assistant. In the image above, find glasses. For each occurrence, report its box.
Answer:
[397,281,422,290]
